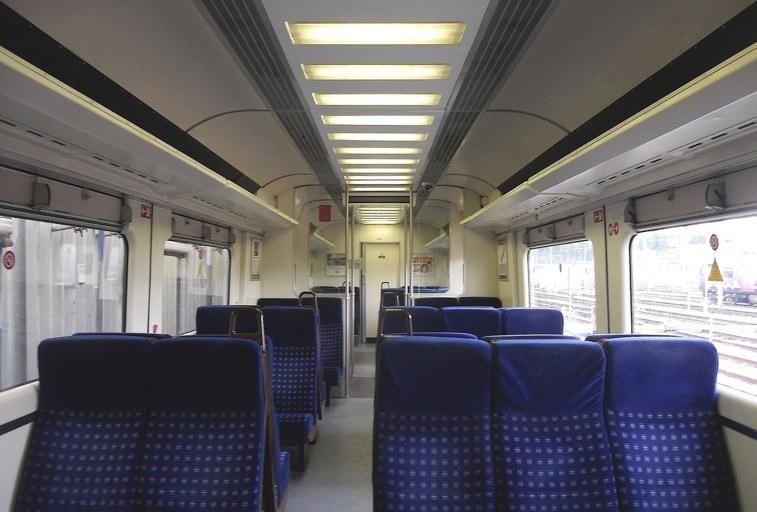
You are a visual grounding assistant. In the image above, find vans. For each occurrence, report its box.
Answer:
[699,262,735,300]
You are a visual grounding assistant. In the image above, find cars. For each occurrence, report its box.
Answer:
[706,276,757,307]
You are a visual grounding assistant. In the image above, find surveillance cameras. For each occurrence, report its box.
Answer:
[421,182,434,194]
